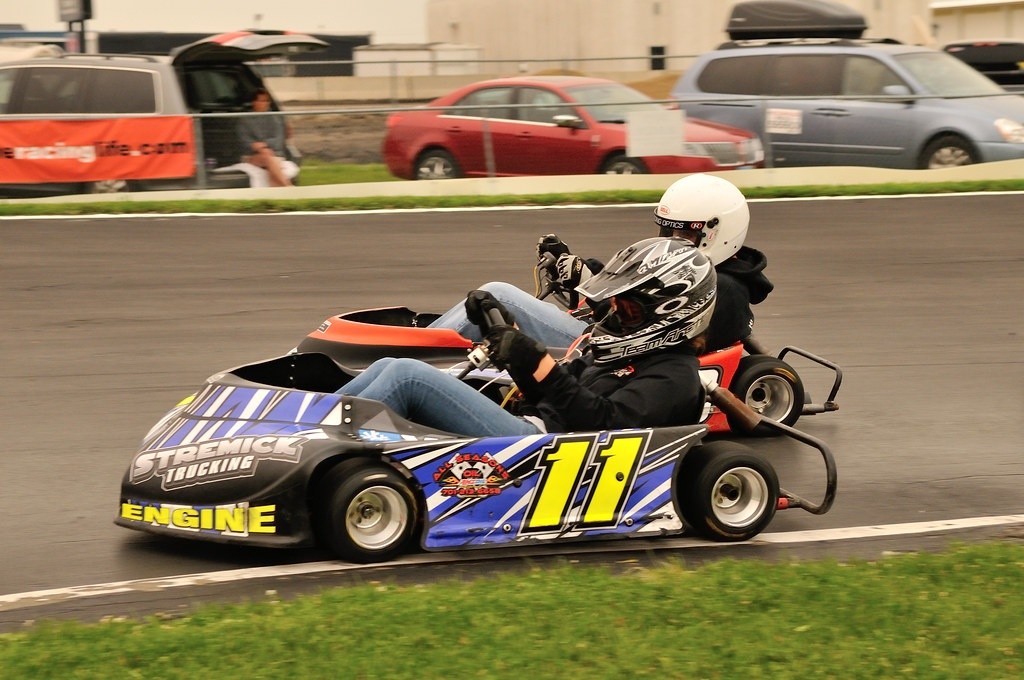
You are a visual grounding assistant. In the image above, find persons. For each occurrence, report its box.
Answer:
[235,88,294,188]
[410,174,778,362]
[326,235,719,434]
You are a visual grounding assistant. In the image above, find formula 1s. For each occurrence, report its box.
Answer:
[108,287,837,565]
[285,232,844,440]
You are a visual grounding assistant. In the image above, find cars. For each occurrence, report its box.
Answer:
[1,26,334,202]
[379,72,765,183]
[670,1,1023,171]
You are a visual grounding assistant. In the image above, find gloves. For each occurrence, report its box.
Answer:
[487,324,548,376]
[465,289,516,328]
[536,234,572,273]
[555,254,595,304]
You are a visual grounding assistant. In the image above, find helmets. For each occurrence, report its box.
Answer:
[654,173,750,268]
[573,235,718,367]
